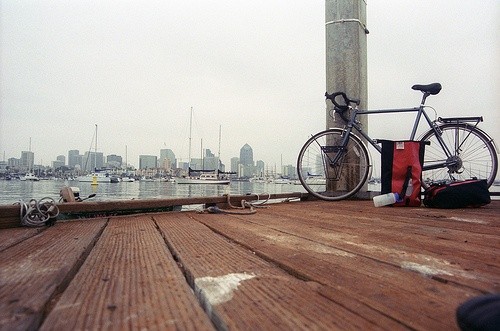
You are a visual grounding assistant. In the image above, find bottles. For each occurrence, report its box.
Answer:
[372,193,403,207]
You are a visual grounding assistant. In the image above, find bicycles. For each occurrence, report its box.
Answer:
[297,83,498,202]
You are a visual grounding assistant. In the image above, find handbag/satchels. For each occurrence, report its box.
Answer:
[422,178,493,209]
[375,139,430,207]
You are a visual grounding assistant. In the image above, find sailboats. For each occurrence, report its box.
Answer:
[0,106,233,186]
